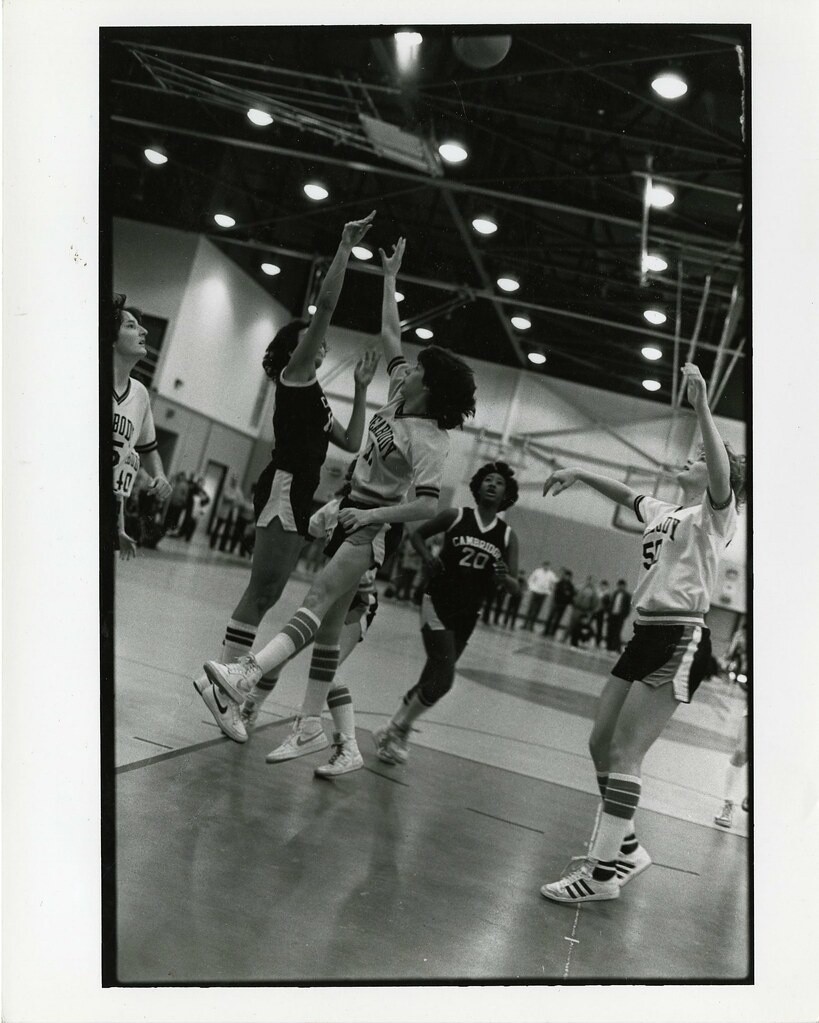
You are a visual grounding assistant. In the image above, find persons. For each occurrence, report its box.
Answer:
[369,460,520,765]
[192,210,479,783]
[292,490,631,655]
[712,622,748,827]
[113,291,257,559]
[538,362,739,902]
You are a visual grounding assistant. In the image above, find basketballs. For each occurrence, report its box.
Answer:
[451,34,511,69]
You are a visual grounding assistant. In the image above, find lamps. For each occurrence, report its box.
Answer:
[643,302,667,324]
[143,27,546,365]
[641,375,661,392]
[651,58,688,101]
[640,341,665,362]
[645,249,669,273]
[646,183,676,212]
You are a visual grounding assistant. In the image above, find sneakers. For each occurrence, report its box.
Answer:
[540,856,620,902]
[742,795,749,812]
[193,674,248,744]
[316,732,364,776]
[386,723,421,764]
[616,844,652,889]
[203,652,262,705]
[715,799,735,827]
[371,724,396,765]
[221,693,264,735]
[266,715,329,764]
